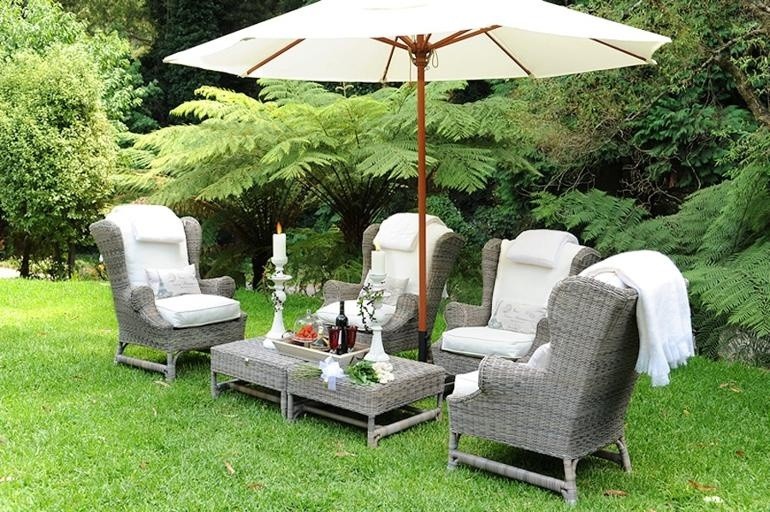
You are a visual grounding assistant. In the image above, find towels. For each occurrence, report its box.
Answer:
[372,212,449,254]
[99,204,184,264]
[507,229,579,269]
[577,250,695,388]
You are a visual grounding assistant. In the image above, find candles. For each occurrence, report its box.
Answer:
[273,222,286,258]
[371,241,385,275]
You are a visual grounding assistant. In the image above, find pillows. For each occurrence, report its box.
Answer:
[358,269,409,305]
[487,296,548,333]
[142,263,201,299]
[527,342,550,370]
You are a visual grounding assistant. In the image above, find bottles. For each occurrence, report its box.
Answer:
[337,301,348,356]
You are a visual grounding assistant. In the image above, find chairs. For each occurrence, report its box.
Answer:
[430,238,601,401]
[89,216,248,382]
[316,223,465,365]
[446,275,689,506]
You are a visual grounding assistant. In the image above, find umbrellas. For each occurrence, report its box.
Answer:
[159,1,676,363]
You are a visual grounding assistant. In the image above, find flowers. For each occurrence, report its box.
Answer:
[288,357,396,391]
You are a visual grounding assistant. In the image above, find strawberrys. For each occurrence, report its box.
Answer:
[297,326,317,338]
